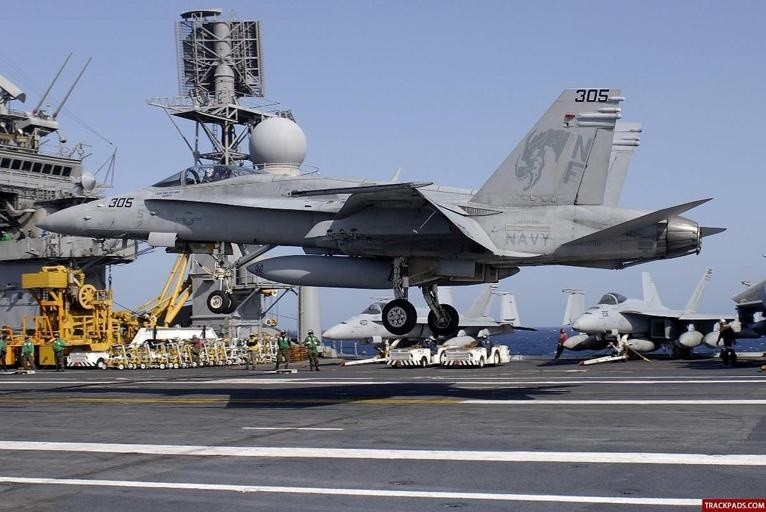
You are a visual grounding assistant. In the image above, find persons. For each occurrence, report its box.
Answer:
[189,334,201,354]
[276,328,290,372]
[20,335,36,371]
[53,331,65,372]
[0,333,7,372]
[421,338,438,356]
[304,329,321,371]
[717,318,738,365]
[552,328,568,360]
[246,331,258,370]
[477,335,495,357]
